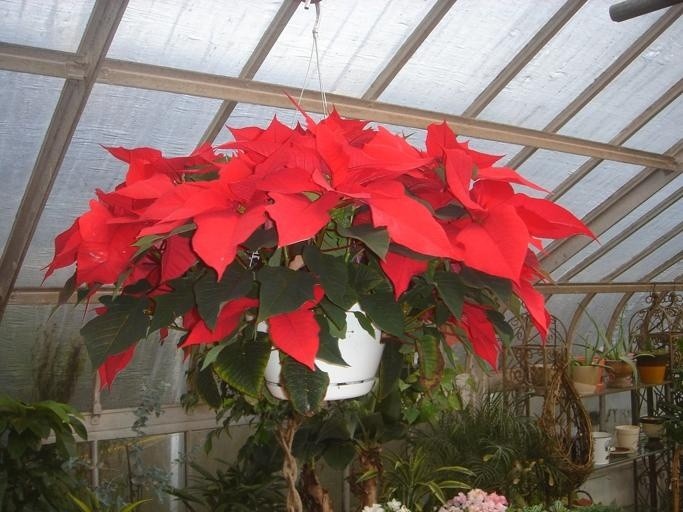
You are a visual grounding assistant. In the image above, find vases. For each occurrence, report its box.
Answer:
[592,416,663,465]
[246,292,384,403]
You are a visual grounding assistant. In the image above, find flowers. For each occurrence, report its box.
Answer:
[43,0,593,416]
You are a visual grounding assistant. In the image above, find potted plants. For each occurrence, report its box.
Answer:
[569,301,671,392]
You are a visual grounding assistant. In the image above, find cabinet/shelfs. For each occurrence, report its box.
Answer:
[503,291,681,510]
